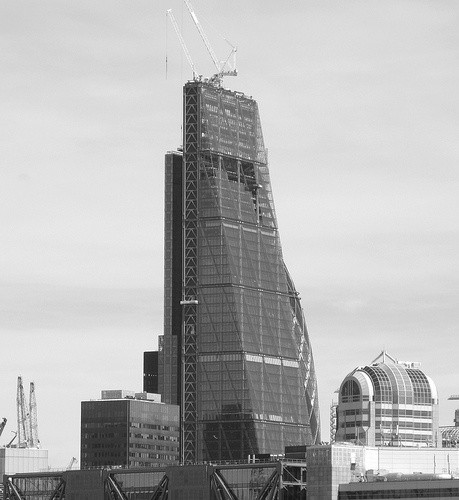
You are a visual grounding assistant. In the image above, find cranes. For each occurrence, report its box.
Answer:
[1,377,38,448]
[167,0,238,87]
